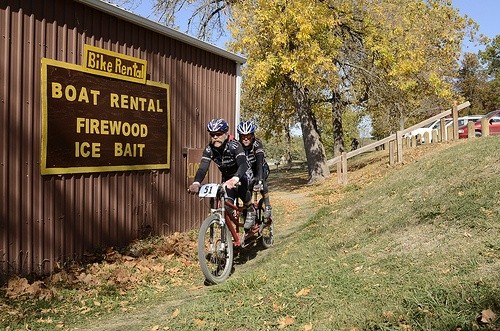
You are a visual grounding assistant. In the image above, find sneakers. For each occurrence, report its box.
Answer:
[244,208,256,229]
[264,205,271,218]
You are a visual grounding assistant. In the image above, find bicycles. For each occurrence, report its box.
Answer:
[187,179,274,286]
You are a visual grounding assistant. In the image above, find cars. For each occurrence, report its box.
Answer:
[432,116,500,143]
[458,110,500,138]
[404,118,453,142]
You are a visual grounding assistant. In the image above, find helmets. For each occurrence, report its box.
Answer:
[237,120,256,135]
[207,118,229,133]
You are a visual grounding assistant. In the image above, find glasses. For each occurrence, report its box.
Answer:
[241,136,252,140]
[210,131,226,136]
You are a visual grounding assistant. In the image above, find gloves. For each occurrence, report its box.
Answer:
[254,182,264,191]
[189,183,199,195]
